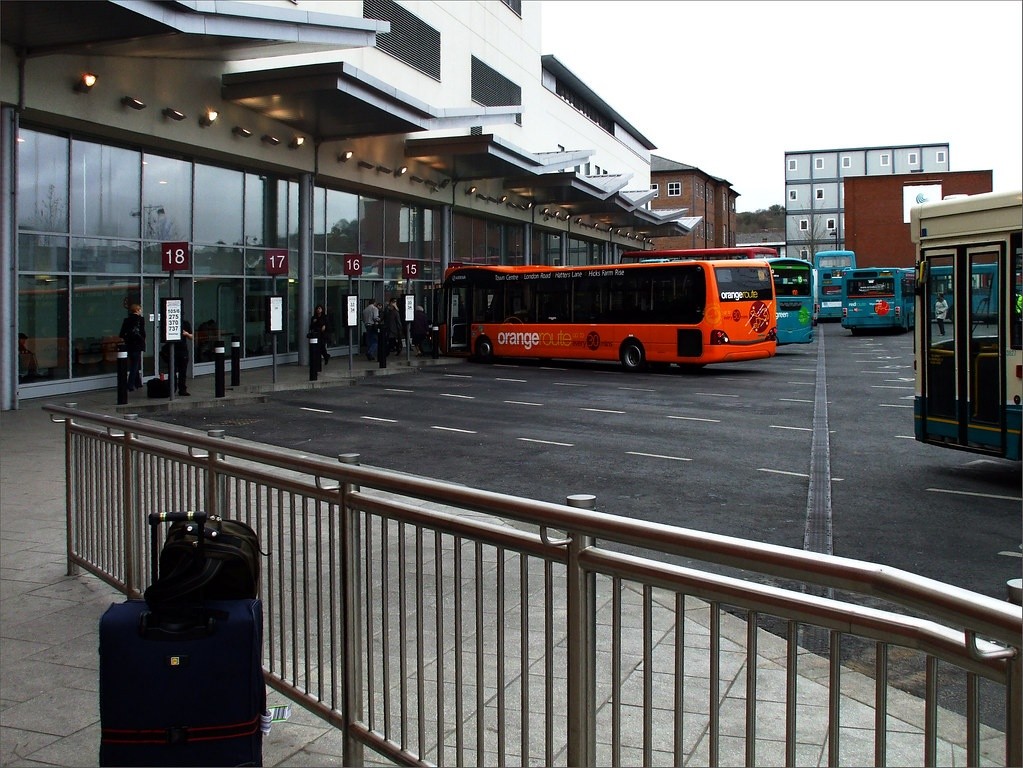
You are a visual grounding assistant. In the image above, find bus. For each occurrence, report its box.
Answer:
[910,191,1023,461]
[620,247,778,260]
[929,263,1023,323]
[440,258,778,373]
[841,267,915,335]
[637,259,814,345]
[814,251,856,321]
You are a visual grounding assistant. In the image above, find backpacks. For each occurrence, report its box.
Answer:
[144,516,259,614]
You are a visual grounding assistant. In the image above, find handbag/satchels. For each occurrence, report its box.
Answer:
[147,378,171,399]
[132,316,145,342]
[161,344,185,367]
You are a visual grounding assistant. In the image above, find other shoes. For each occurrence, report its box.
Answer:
[324,354,330,365]
[937,333,943,336]
[418,338,433,356]
[179,392,191,396]
[366,352,376,361]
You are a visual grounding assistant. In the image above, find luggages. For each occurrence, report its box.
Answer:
[99,512,264,768]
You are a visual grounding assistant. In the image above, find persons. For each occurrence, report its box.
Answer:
[311,305,330,365]
[362,298,427,361]
[173,314,192,396]
[119,304,146,391]
[18,332,27,351]
[1015,290,1023,315]
[935,291,948,336]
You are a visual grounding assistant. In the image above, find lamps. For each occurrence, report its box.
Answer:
[440,179,450,190]
[395,166,408,177]
[261,135,280,145]
[378,165,391,175]
[476,193,488,200]
[398,273,413,284]
[161,107,187,121]
[490,196,497,203]
[411,176,423,184]
[288,275,294,283]
[465,186,476,195]
[122,97,146,109]
[232,126,252,137]
[358,160,375,169]
[289,136,305,149]
[338,150,353,163]
[424,179,437,187]
[198,111,221,126]
[499,194,652,244]
[72,73,97,95]
